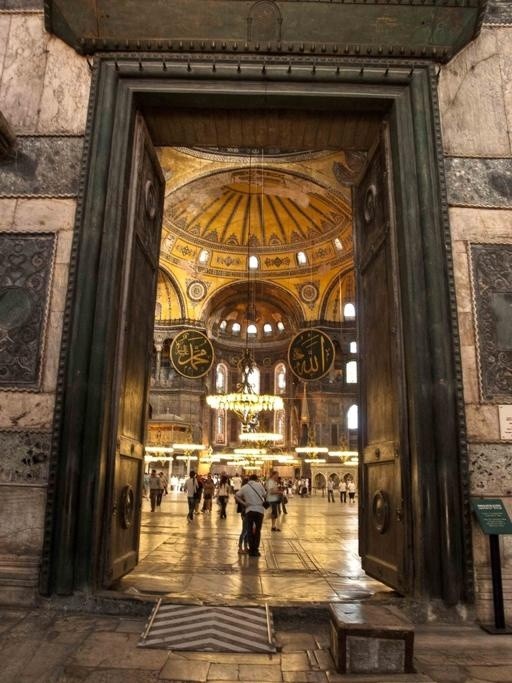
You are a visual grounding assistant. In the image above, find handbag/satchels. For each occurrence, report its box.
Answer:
[262,499,272,510]
[283,495,287,504]
[223,498,230,503]
[193,492,200,501]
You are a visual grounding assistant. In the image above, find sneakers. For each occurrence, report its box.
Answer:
[186,508,227,522]
[271,526,281,531]
[236,546,261,556]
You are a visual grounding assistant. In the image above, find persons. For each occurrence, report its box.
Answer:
[143,467,358,556]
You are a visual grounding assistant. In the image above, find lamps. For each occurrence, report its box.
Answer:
[204,192,286,426]
